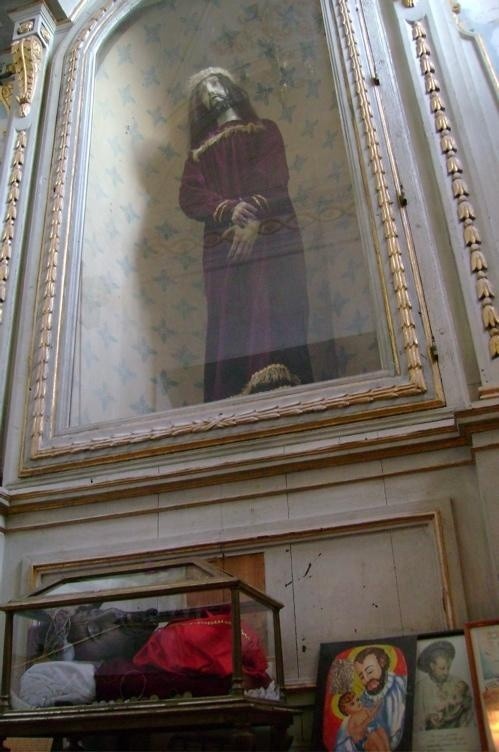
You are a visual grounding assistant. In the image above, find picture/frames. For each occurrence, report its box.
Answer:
[410,628,485,751]
[465,619,499,752]
[310,634,422,751]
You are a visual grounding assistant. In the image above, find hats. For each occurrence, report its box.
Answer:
[418,640,458,673]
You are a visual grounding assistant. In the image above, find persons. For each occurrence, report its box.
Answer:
[334,641,474,752]
[178,66,316,398]
